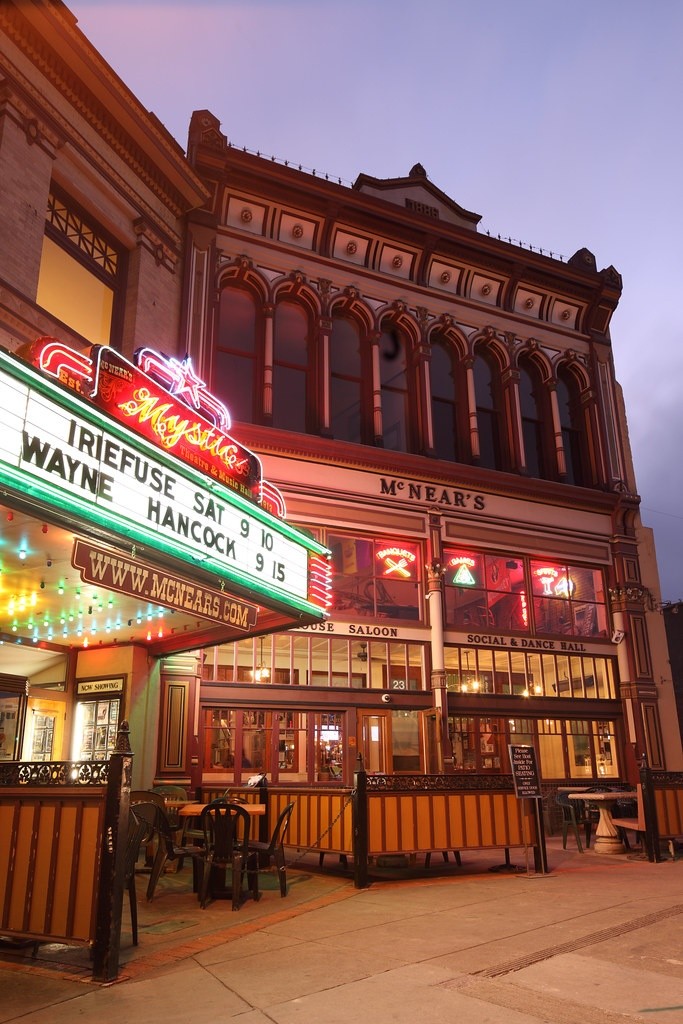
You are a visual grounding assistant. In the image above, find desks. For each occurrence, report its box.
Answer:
[178,804,266,815]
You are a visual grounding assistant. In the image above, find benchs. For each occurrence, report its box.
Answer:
[569,792,638,854]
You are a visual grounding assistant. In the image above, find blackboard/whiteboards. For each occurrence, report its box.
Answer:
[507,744,543,799]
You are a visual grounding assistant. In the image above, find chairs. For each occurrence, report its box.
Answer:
[124,786,293,946]
[557,785,630,853]
